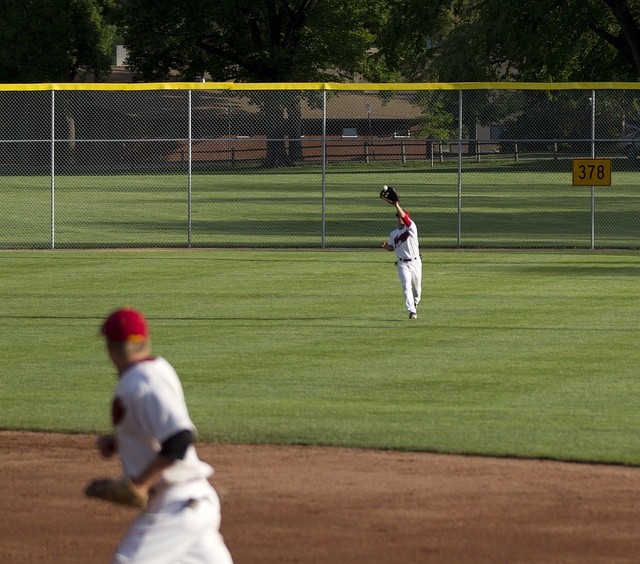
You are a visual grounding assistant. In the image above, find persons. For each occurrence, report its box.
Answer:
[379,186,422,319]
[83,306,235,563]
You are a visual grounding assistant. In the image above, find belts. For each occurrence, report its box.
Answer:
[399,257,416,262]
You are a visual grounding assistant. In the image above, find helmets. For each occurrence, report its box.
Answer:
[395,210,411,220]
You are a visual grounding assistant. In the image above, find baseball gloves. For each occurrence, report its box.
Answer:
[85,476,147,509]
[380,186,398,205]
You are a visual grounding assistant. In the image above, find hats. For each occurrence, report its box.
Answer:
[101,305,150,344]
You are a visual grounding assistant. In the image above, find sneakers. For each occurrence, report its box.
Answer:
[409,311,418,319]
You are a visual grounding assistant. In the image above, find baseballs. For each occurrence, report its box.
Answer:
[384,185,389,192]
